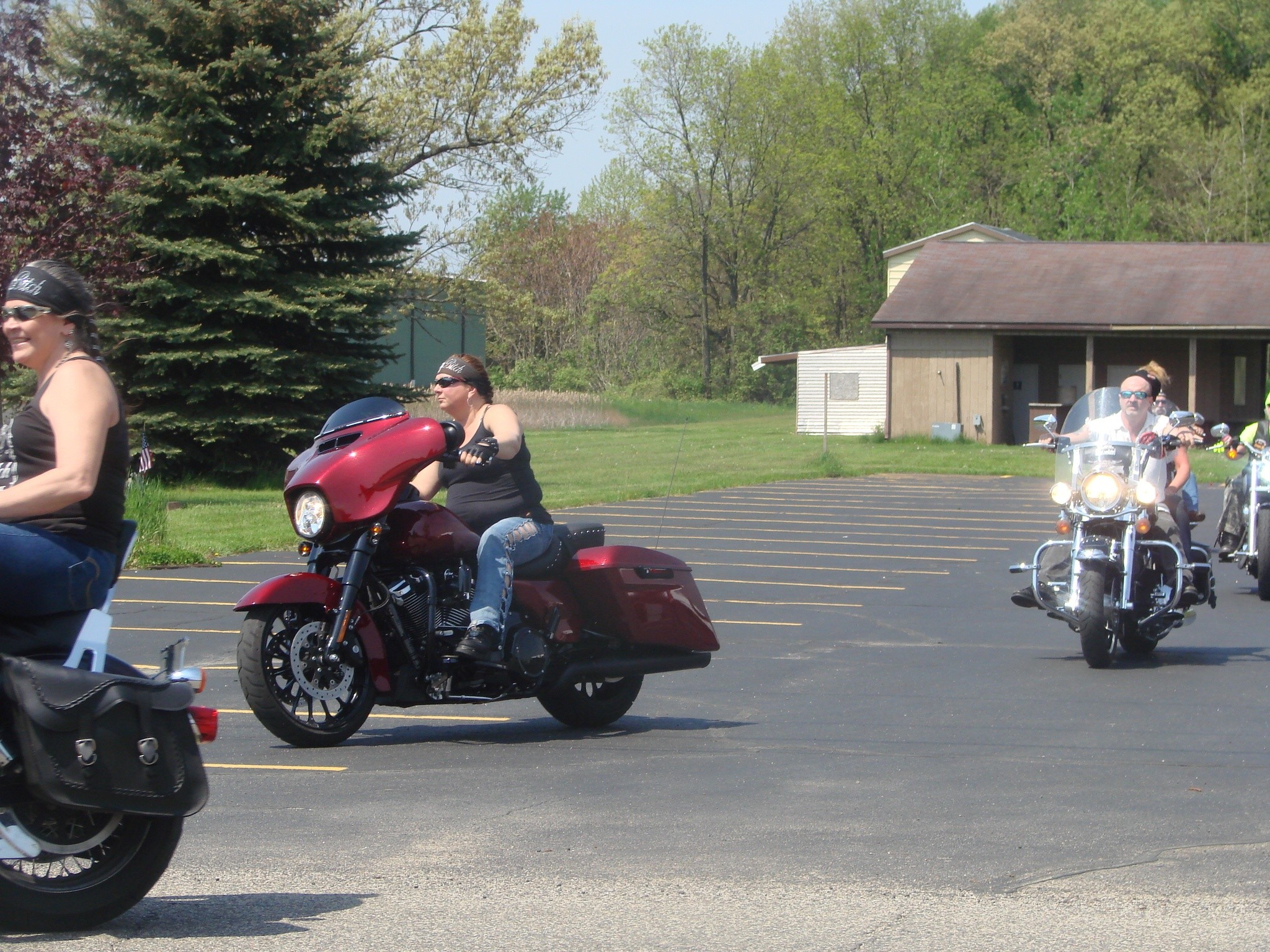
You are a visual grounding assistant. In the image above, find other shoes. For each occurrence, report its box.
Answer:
[1216,532,1238,559]
[456,625,486,661]
[1181,585,1199,605]
[1011,580,1058,611]
[1189,510,1205,522]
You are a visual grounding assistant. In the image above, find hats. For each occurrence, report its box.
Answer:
[1158,389,1167,397]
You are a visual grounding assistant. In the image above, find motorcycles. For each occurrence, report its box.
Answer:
[1205,422,1270,602]
[0,518,218,935]
[1009,386,1212,669]
[234,398,721,748]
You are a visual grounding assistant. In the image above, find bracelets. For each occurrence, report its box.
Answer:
[480,436,499,457]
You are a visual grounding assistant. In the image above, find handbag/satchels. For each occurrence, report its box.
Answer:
[1,651,211,819]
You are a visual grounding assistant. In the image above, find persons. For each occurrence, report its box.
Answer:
[1010,361,1205,611]
[407,353,554,657]
[0,258,127,618]
[1216,392,1270,558]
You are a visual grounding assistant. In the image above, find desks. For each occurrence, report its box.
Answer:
[1028,402,1072,445]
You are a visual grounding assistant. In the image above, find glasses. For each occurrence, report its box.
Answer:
[0,306,52,322]
[1156,400,1167,404]
[1119,391,1150,400]
[434,377,458,388]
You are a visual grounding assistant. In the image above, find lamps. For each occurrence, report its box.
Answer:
[751,356,767,372]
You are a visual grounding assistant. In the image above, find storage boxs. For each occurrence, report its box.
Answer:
[569,546,721,653]
